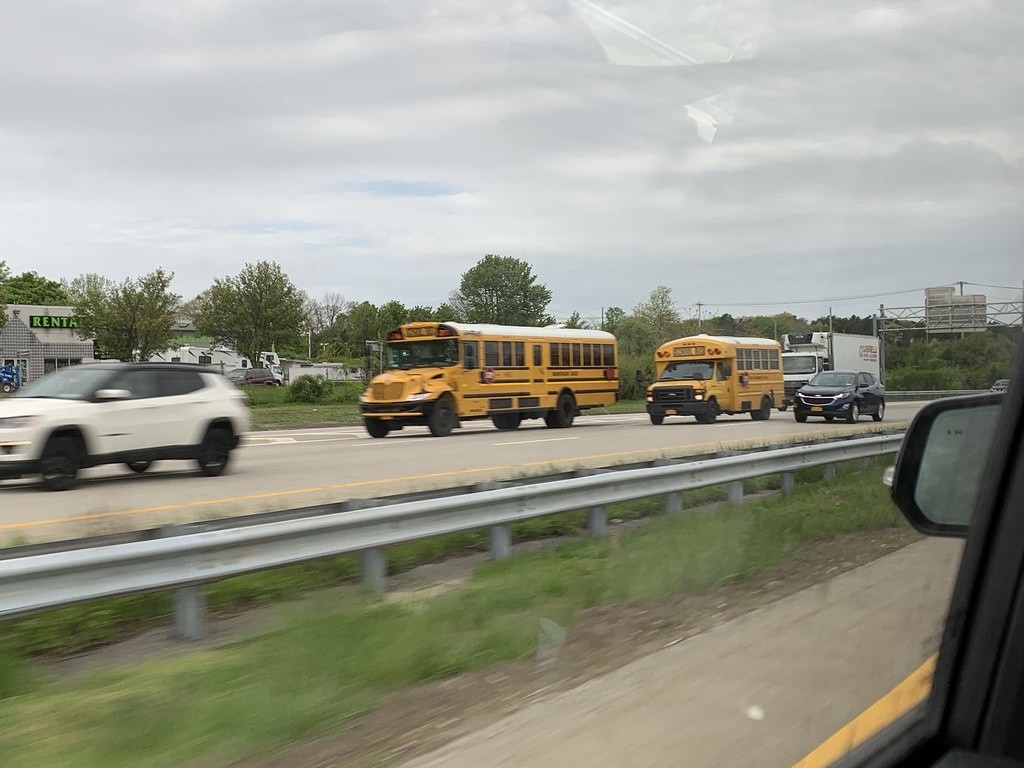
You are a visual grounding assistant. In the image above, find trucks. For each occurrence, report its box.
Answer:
[778,333,879,412]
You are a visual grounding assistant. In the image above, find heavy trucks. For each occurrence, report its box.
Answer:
[180,347,284,387]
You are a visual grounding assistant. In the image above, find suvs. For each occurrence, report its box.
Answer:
[223,368,277,387]
[794,370,887,424]
[0,360,252,486]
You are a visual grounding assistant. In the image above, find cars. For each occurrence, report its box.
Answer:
[991,380,1010,393]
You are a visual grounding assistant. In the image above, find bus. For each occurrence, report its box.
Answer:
[645,336,785,423]
[356,320,617,437]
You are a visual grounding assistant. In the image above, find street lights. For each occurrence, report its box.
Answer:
[320,343,329,351]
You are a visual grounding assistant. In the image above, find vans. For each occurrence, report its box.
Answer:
[0,366,19,393]
